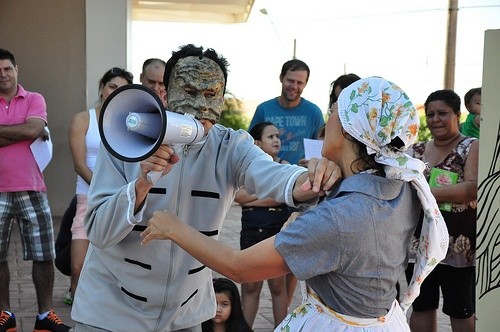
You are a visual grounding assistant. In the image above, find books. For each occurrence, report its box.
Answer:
[428,167,458,211]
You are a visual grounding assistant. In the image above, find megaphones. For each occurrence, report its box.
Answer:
[99,85,205,184]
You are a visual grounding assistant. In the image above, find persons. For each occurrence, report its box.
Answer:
[55,195,78,304]
[235,121,291,329]
[405,90,479,332]
[71,67,134,305]
[202,278,254,332]
[141,75,449,332]
[139,58,168,110]
[70,44,343,332]
[318,73,361,140]
[248,59,324,305]
[0,47,70,332]
[461,88,481,140]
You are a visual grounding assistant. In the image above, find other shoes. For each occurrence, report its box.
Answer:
[64,288,73,306]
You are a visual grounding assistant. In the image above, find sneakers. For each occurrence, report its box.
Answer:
[0,310,17,332]
[33,310,73,332]
